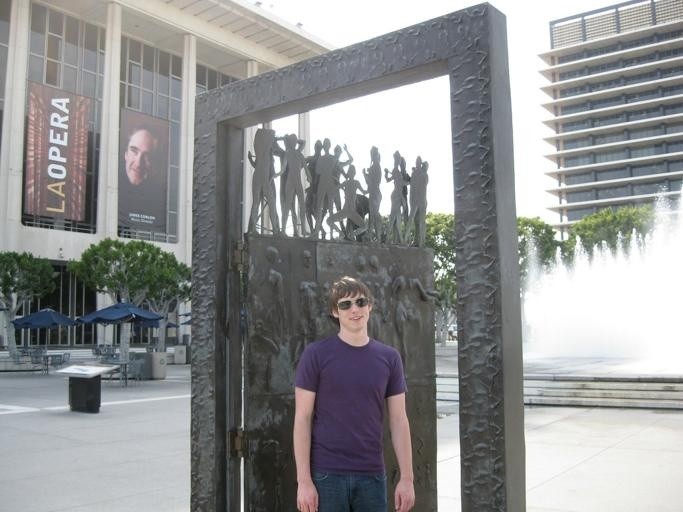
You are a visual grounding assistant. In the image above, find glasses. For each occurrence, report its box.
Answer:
[336,297,369,310]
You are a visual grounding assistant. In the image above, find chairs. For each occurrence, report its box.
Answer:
[92,344,145,388]
[20,346,70,377]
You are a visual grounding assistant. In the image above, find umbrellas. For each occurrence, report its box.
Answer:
[9,303,191,353]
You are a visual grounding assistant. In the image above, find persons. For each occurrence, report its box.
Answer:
[293,275,416,512]
[248,128,428,247]
[118,127,166,226]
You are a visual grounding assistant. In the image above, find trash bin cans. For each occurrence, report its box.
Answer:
[175,345,186,364]
[136,352,166,379]
